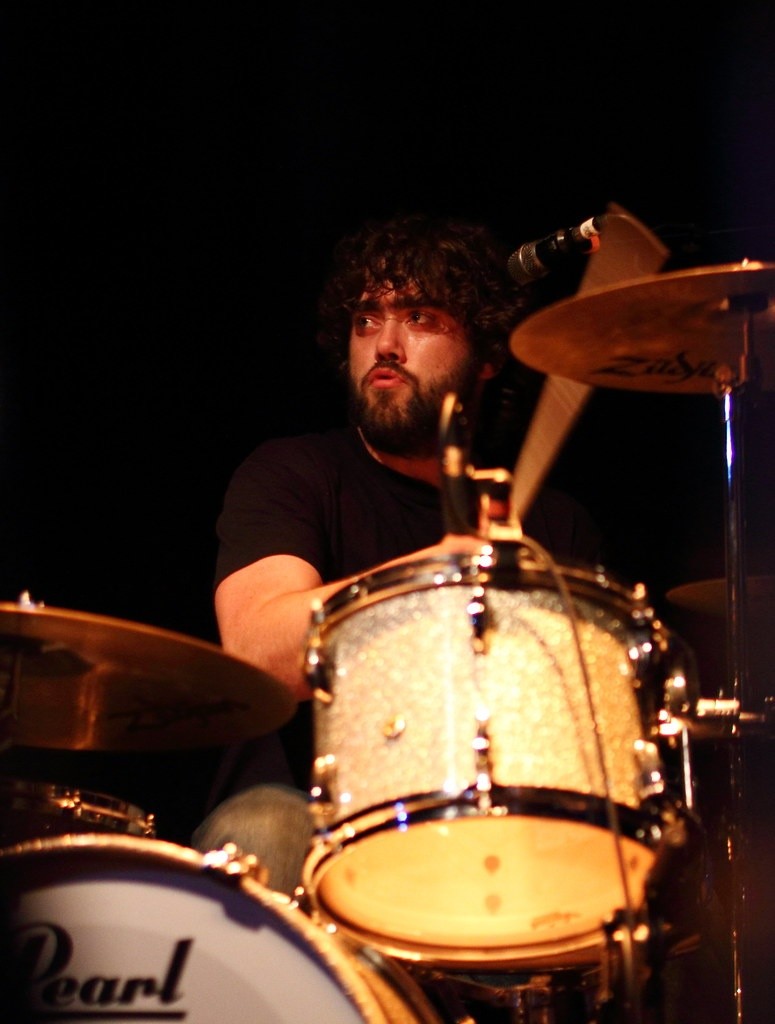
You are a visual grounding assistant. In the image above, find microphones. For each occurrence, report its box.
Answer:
[507,214,609,286]
[440,401,476,536]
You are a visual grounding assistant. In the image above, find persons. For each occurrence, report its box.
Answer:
[191,210,603,900]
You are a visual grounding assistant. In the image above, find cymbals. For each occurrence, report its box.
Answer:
[664,574,775,624]
[0,600,299,755]
[507,259,775,397]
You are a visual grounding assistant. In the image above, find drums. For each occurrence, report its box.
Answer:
[301,549,697,968]
[0,819,444,1024]
[0,779,156,850]
[656,691,775,905]
